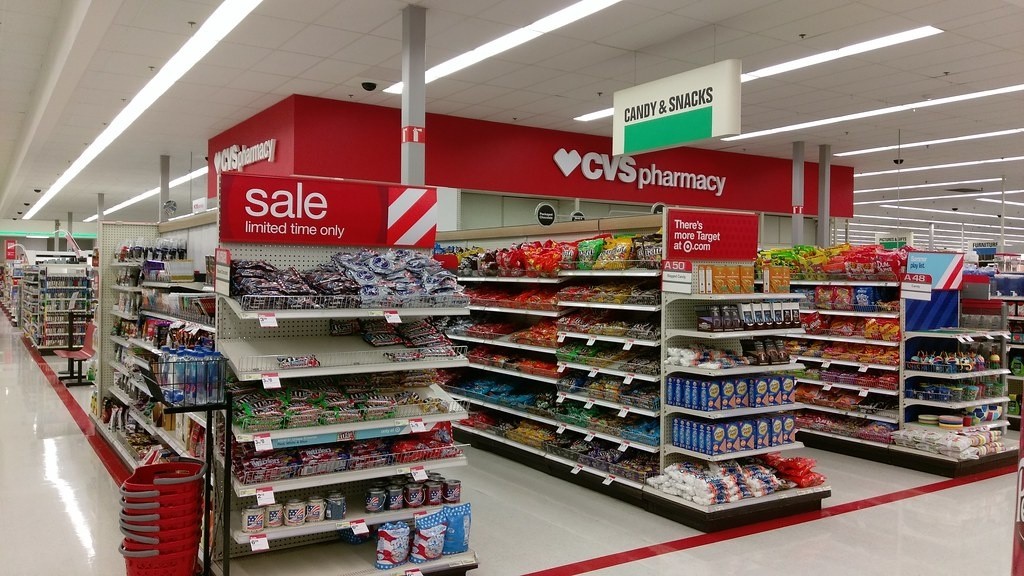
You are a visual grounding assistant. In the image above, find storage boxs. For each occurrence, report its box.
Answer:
[667,375,795,412]
[698,265,755,295]
[672,415,796,456]
[1006,321,1024,344]
[1009,301,1024,317]
[740,336,791,365]
[694,302,801,333]
[762,265,791,294]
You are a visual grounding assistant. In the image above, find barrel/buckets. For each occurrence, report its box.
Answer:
[242,490,347,533]
[364,471,463,512]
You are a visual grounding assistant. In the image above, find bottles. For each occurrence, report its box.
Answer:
[916,382,1003,400]
[158,345,224,406]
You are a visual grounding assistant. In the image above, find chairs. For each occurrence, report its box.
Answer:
[53,324,97,388]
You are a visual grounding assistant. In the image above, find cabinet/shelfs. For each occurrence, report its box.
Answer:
[758,252,1020,478]
[435,206,831,533]
[100,172,480,576]
[0,243,93,356]
[991,284,1024,431]
